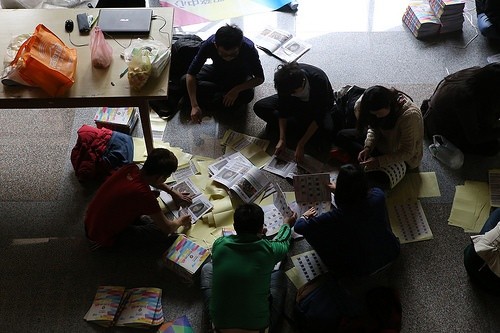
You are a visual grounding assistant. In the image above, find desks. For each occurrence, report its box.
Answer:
[0,8,175,154]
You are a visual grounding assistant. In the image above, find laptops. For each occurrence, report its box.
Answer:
[98,8,152,34]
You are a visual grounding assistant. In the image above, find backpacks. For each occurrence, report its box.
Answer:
[170,35,207,79]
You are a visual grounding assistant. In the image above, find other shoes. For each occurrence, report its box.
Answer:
[177,96,190,106]
[330,147,352,164]
[258,126,266,139]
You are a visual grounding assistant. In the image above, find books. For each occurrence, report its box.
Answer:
[166,236,211,275]
[364,157,407,188]
[255,28,312,64]
[83,284,165,332]
[154,129,326,225]
[402,0,465,39]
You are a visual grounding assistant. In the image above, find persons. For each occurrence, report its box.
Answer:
[205,202,298,333]
[463,209,500,296]
[293,165,402,288]
[181,25,265,123]
[476,0,500,41]
[252,61,334,165]
[426,63,500,171]
[333,85,424,191]
[83,148,193,255]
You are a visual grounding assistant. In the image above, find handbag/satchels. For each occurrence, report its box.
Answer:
[2,32,35,87]
[124,37,172,90]
[330,86,367,132]
[293,276,348,333]
[11,23,76,100]
[89,26,112,68]
[428,135,464,169]
[75,130,135,189]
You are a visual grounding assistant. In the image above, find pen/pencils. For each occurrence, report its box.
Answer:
[120,68,128,78]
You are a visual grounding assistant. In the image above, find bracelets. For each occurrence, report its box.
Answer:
[167,187,173,193]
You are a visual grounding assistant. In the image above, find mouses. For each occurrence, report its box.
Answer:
[65,19,74,32]
